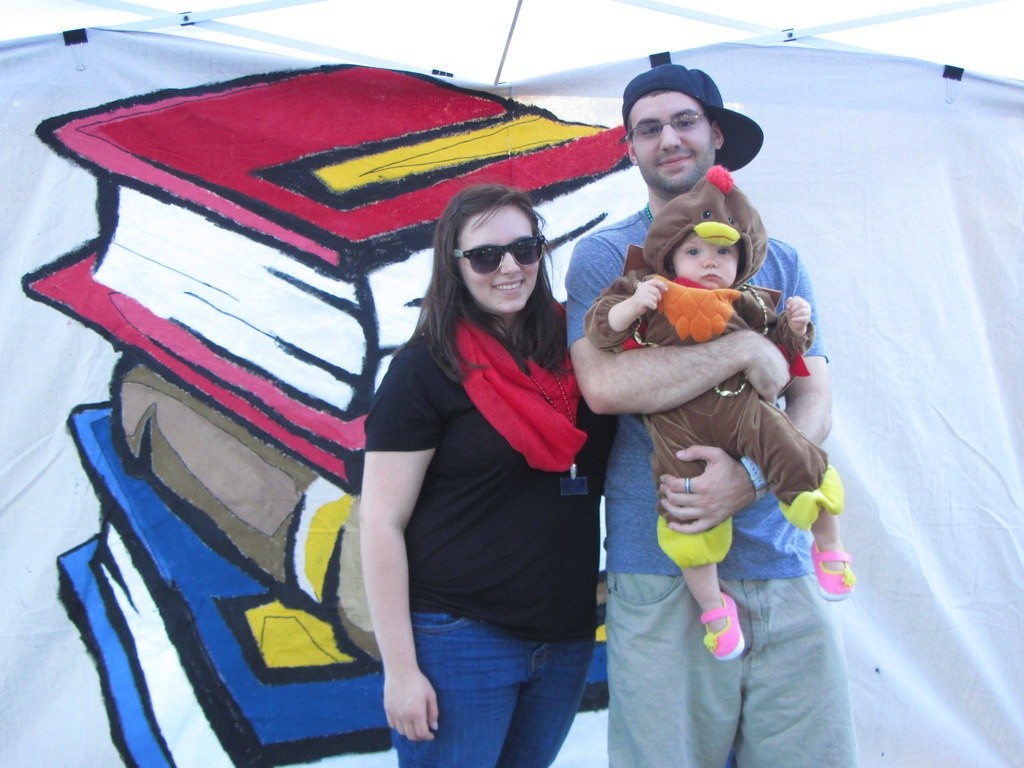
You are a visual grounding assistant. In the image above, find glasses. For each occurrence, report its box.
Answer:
[628,111,709,143]
[454,236,545,274]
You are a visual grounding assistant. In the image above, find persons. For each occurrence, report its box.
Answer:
[564,64,863,768]
[359,188,618,768]
[584,165,856,661]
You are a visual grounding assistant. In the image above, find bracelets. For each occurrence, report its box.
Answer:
[740,454,768,501]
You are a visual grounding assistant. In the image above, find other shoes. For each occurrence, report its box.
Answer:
[700,592,745,661]
[810,540,856,602]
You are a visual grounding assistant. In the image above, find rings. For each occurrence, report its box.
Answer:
[684,477,691,493]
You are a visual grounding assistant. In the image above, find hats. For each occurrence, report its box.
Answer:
[621,64,764,173]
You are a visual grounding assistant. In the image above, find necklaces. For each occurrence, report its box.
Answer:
[529,320,578,480]
[645,201,655,223]
[632,283,769,397]
